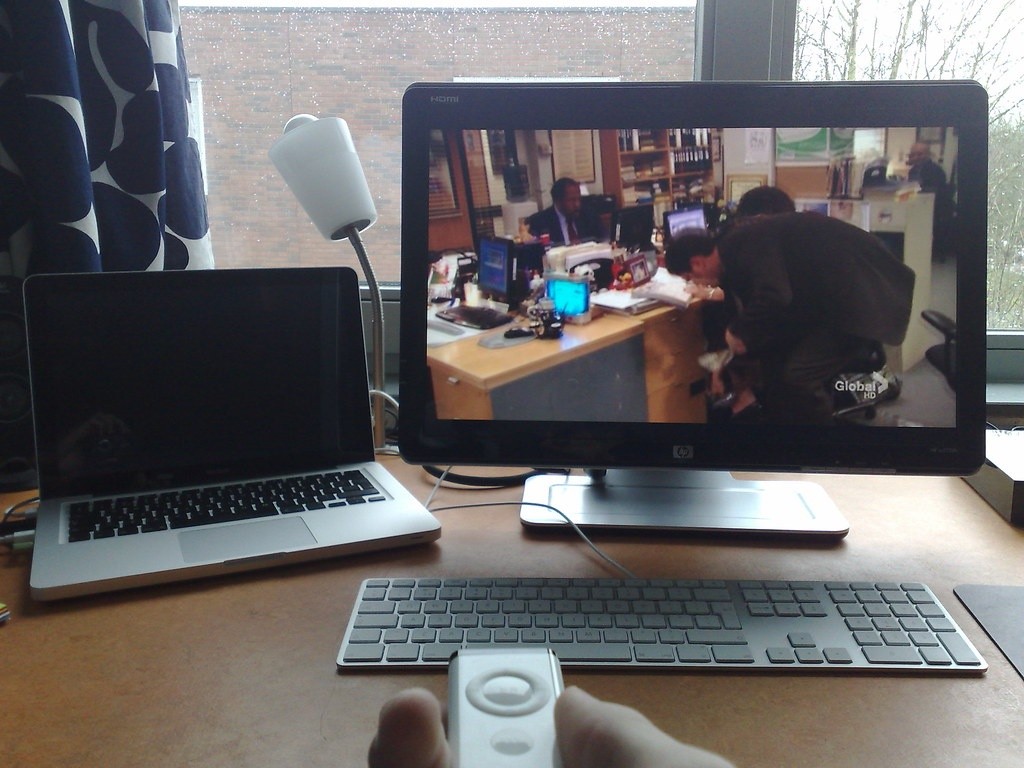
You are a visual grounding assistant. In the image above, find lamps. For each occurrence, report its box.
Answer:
[268,115,389,455]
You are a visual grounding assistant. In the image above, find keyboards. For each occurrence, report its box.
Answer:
[436,304,512,329]
[336,578,989,673]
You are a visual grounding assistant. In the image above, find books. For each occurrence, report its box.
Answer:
[546,241,616,293]
[618,128,713,209]
[632,264,693,309]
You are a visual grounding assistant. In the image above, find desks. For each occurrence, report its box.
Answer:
[0,447,1024,768]
[427,304,710,422]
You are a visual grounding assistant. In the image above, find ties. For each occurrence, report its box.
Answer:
[565,217,578,244]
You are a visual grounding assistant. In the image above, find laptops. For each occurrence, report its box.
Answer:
[23,267,443,603]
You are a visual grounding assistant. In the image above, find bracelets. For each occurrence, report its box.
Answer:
[709,289,715,299]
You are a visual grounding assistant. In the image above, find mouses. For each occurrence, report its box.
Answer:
[504,326,534,338]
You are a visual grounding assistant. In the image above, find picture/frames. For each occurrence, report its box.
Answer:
[916,127,947,144]
[548,130,597,184]
[624,255,650,287]
[726,174,768,202]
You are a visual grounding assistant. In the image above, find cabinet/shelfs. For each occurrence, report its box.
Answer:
[598,128,715,227]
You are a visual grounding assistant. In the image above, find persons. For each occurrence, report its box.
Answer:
[368,685,735,768]
[910,142,949,262]
[525,175,616,247]
[685,183,796,304]
[661,207,915,425]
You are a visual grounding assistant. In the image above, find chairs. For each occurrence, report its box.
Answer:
[919,309,960,391]
[908,163,958,261]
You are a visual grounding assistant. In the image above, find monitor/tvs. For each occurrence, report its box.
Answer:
[663,205,707,239]
[398,78,989,538]
[609,203,654,253]
[477,234,517,311]
